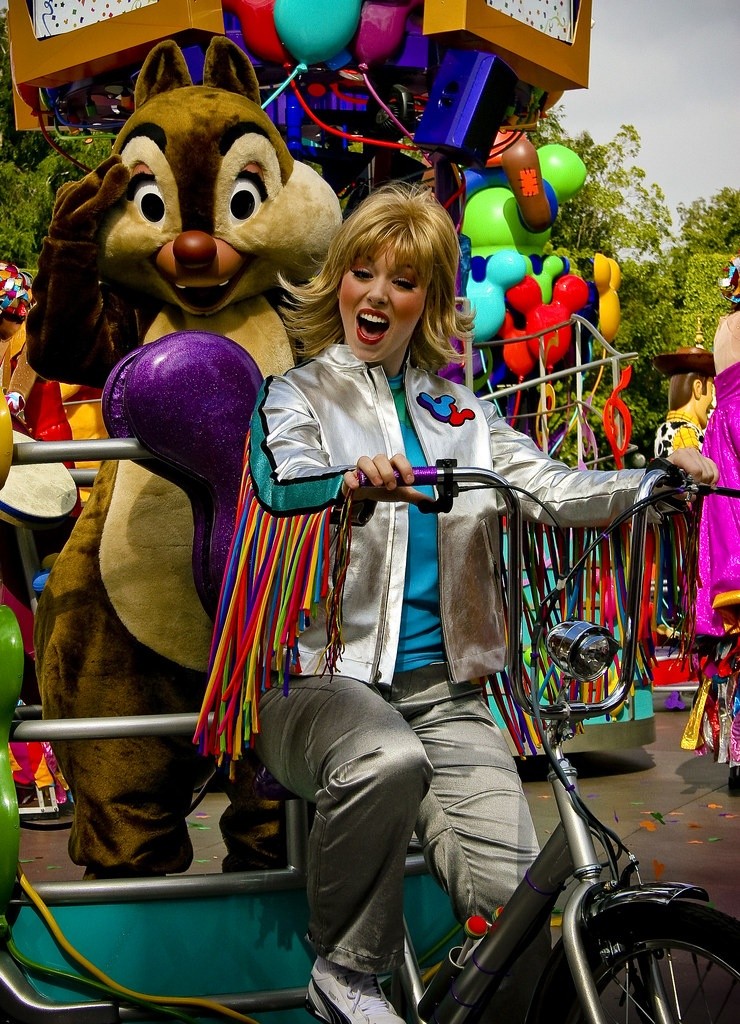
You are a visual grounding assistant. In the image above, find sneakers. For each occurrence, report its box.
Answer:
[306,958,407,1024]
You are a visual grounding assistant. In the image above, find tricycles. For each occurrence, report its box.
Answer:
[0,432,740,1024]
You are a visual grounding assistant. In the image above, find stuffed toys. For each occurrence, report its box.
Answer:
[27,32,344,884]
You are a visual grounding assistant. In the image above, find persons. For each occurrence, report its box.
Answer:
[253,184,721,1024]
[654,346,716,481]
